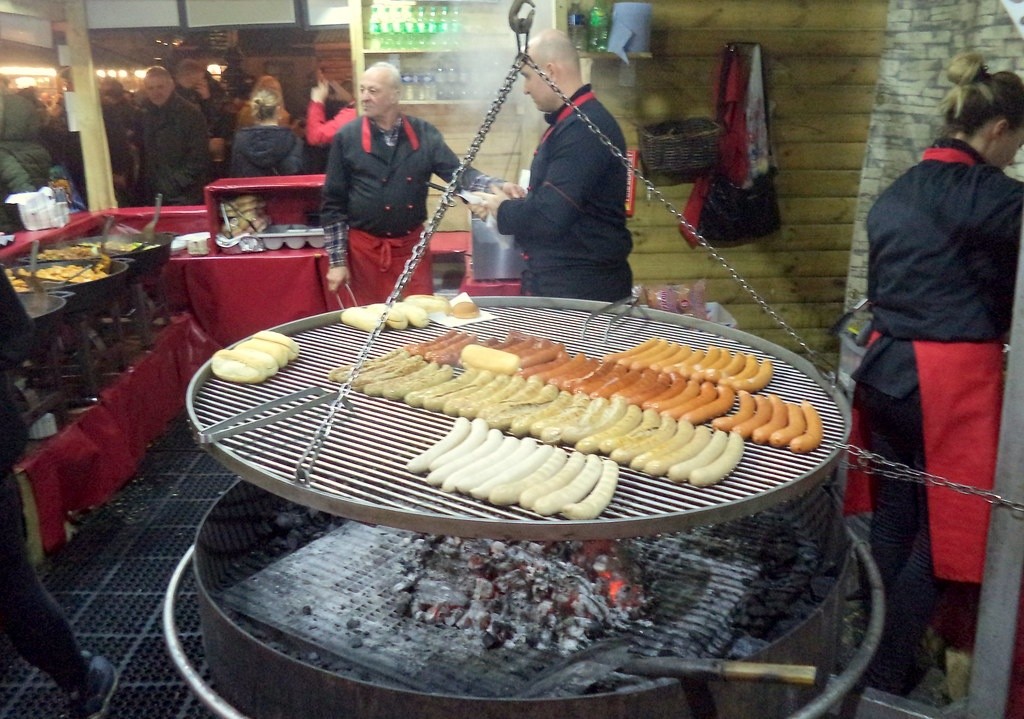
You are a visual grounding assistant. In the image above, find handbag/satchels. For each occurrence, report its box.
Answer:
[697,167,780,247]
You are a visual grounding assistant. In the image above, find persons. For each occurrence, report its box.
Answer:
[0,59,358,234]
[849,49,1024,696]
[467,30,633,304]
[0,266,119,719]
[320,62,524,307]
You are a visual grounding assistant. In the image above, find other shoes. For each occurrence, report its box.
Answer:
[63,650,117,719]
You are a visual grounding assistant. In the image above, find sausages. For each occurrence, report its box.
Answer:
[330,330,822,521]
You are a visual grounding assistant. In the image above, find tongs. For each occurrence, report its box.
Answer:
[579,294,639,340]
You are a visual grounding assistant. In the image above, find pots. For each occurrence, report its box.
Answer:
[0,227,179,364]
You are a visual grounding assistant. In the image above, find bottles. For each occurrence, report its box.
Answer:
[588,0,613,52]
[566,1,593,55]
[365,3,513,102]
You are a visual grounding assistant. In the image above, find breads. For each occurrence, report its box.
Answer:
[210,331,301,383]
[342,294,482,332]
[219,194,269,237]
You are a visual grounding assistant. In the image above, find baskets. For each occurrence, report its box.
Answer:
[637,117,724,175]
